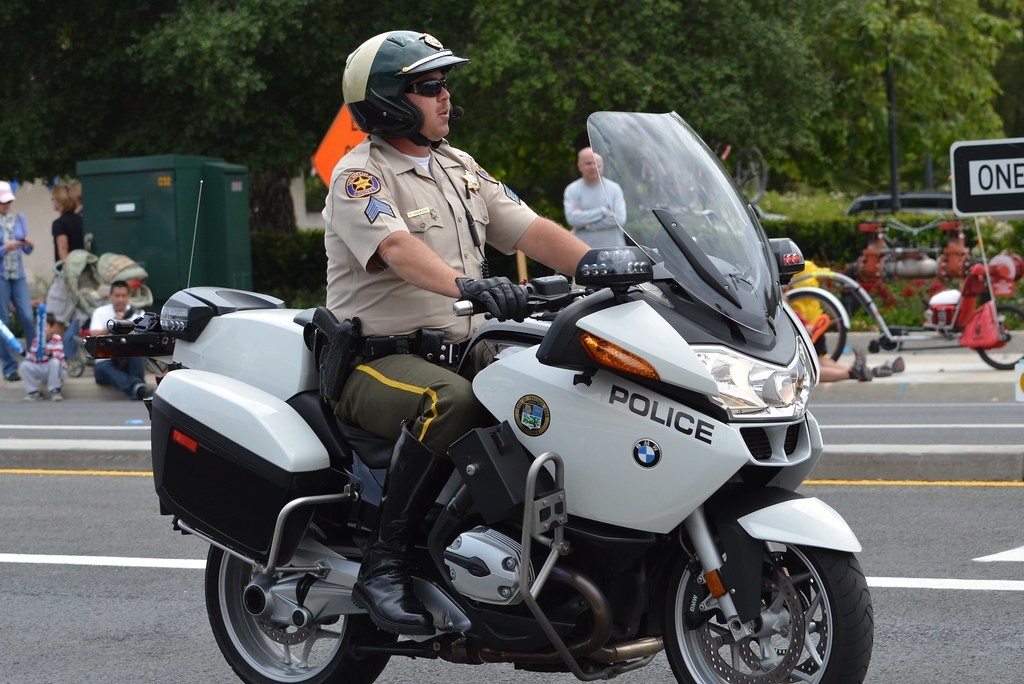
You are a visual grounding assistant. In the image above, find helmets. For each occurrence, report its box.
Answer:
[343,31,471,139]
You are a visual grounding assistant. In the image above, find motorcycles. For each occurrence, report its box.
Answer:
[84,112,874,684]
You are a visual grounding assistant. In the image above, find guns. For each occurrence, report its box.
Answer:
[312,306,357,352]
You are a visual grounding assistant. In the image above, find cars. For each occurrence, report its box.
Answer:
[846,190,955,219]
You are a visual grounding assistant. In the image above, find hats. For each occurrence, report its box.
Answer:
[0,181,15,203]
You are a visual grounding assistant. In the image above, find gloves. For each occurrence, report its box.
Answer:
[455,277,531,323]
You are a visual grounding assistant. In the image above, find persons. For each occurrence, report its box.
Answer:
[90,280,159,400]
[19,314,68,401]
[564,148,627,248]
[0,181,36,380]
[50,181,84,337]
[789,262,904,383]
[313,32,593,633]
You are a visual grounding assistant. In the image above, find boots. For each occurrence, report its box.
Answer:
[353,426,437,635]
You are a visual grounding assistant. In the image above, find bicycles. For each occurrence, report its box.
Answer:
[785,213,1024,370]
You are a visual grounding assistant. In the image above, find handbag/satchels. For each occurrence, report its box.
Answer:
[45,259,78,322]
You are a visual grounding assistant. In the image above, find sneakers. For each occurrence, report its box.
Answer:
[872,357,904,377]
[849,347,872,382]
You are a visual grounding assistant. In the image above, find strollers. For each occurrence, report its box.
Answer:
[54,248,153,379]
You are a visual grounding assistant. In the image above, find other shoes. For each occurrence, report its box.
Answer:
[134,385,150,400]
[50,390,63,402]
[4,367,21,380]
[23,391,43,402]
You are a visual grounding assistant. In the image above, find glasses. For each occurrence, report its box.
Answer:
[405,78,448,97]
